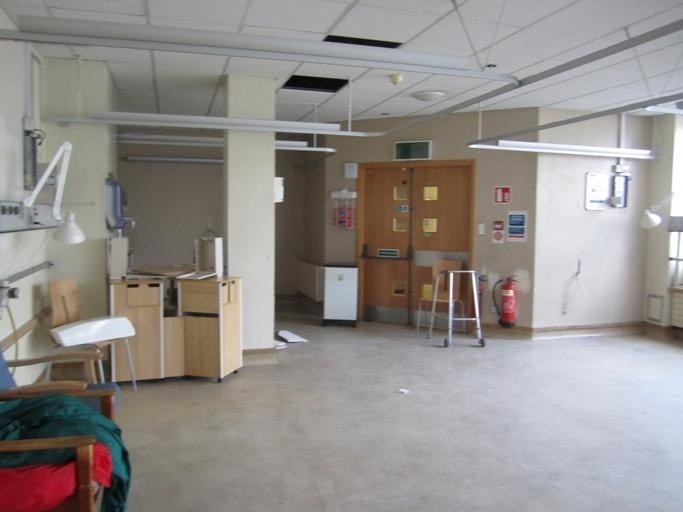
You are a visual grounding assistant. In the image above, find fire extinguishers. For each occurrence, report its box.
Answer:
[492,273,518,328]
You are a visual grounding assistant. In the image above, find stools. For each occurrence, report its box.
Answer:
[427,268,484,350]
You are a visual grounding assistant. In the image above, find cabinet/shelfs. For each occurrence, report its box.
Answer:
[109,265,241,386]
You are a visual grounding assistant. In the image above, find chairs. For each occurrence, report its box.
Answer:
[414,260,466,337]
[0,341,120,418]
[0,385,130,512]
[47,280,138,395]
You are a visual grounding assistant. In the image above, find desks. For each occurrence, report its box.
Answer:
[132,268,198,311]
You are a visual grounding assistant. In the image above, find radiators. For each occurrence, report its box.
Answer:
[294,258,323,303]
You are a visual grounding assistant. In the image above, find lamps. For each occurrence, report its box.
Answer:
[639,189,682,231]
[47,48,387,138]
[22,140,84,247]
[117,145,292,165]
[411,89,445,104]
[474,92,653,160]
[112,53,338,157]
